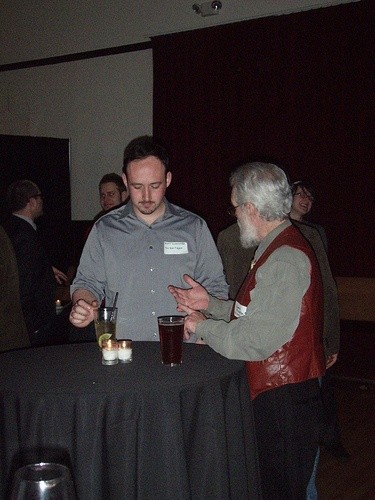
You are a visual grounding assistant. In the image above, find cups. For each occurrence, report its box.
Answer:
[157,315,185,367]
[9,462,77,500]
[93,307,118,351]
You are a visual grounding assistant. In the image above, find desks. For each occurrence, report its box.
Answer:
[0,340,264,500]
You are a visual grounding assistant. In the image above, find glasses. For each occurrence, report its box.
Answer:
[227,204,243,216]
[33,194,45,200]
[294,193,314,202]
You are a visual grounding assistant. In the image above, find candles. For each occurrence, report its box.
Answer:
[103,339,132,360]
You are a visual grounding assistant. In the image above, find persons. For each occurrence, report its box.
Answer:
[68,135,229,344]
[289,179,329,256]
[5,181,69,347]
[167,162,326,500]
[215,220,339,370]
[92,173,129,223]
